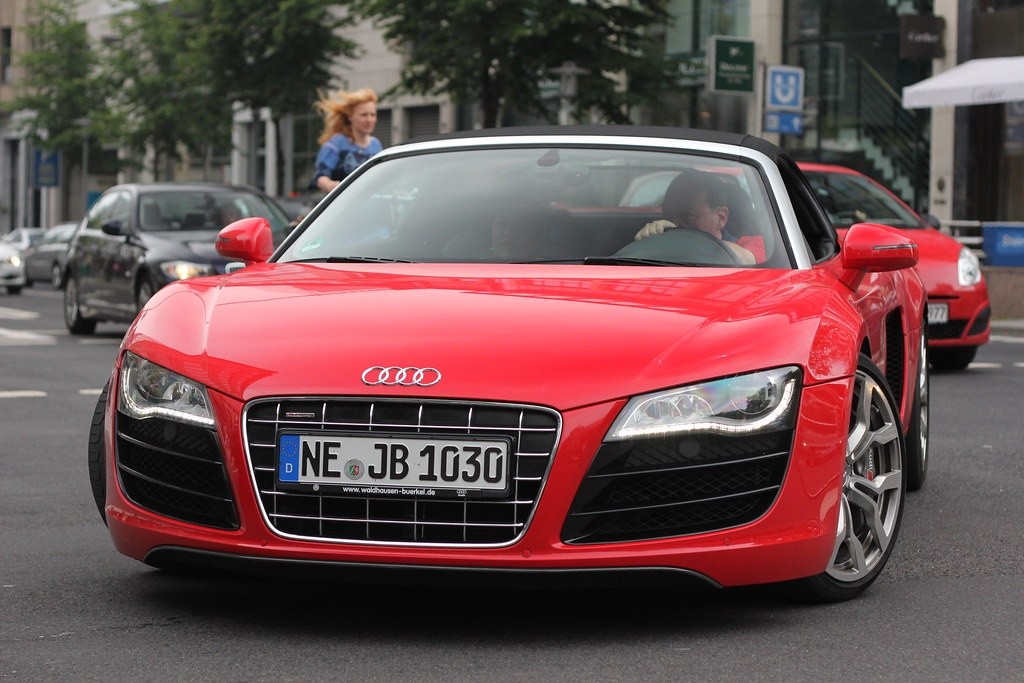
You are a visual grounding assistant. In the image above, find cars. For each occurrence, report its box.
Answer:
[0,242,27,293]
[614,159,994,364]
[25,222,84,290]
[59,181,298,337]
[1,226,49,260]
[85,122,937,604]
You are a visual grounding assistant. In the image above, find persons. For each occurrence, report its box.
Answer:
[309,87,386,193]
[634,169,757,266]
